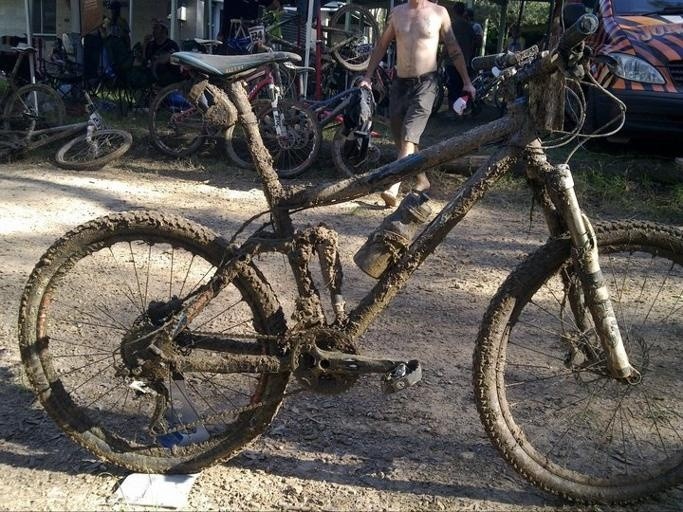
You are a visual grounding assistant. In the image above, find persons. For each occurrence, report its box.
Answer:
[141,18,180,89]
[437,3,484,118]
[358,0,476,207]
[504,14,521,52]
[81,2,131,83]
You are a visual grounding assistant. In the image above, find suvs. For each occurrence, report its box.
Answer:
[552,0,682,155]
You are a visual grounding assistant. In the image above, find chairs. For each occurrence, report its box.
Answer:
[1,34,177,118]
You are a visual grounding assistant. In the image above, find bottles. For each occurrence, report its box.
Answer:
[353,193,433,278]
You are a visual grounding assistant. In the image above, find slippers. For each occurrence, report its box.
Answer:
[381,191,395,207]
[409,188,431,193]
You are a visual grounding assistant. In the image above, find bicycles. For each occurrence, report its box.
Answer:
[14,18,681,504]
